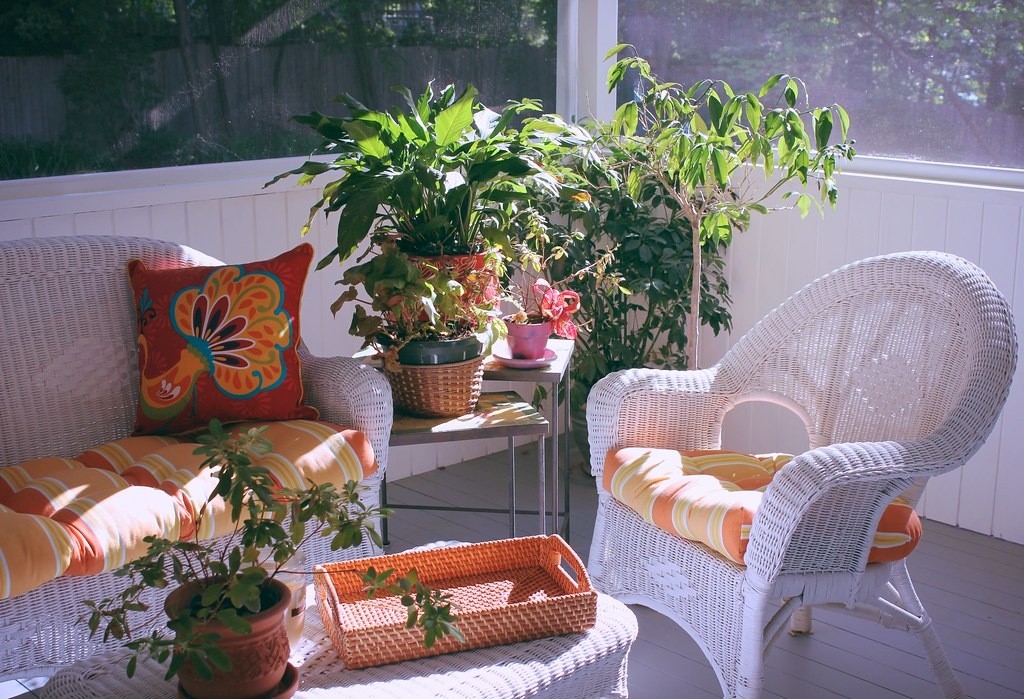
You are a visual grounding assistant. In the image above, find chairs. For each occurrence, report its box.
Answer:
[582,249,1020,697]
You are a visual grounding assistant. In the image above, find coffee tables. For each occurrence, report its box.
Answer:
[37,531,642,699]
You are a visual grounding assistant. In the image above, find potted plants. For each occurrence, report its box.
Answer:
[484,206,626,371]
[73,416,464,699]
[262,77,564,352]
[542,108,736,484]
[327,242,514,417]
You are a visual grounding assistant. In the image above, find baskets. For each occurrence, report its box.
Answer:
[313,533,598,670]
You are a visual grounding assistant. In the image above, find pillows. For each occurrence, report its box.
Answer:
[121,242,322,433]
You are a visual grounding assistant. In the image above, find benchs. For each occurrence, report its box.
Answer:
[0,234,396,693]
